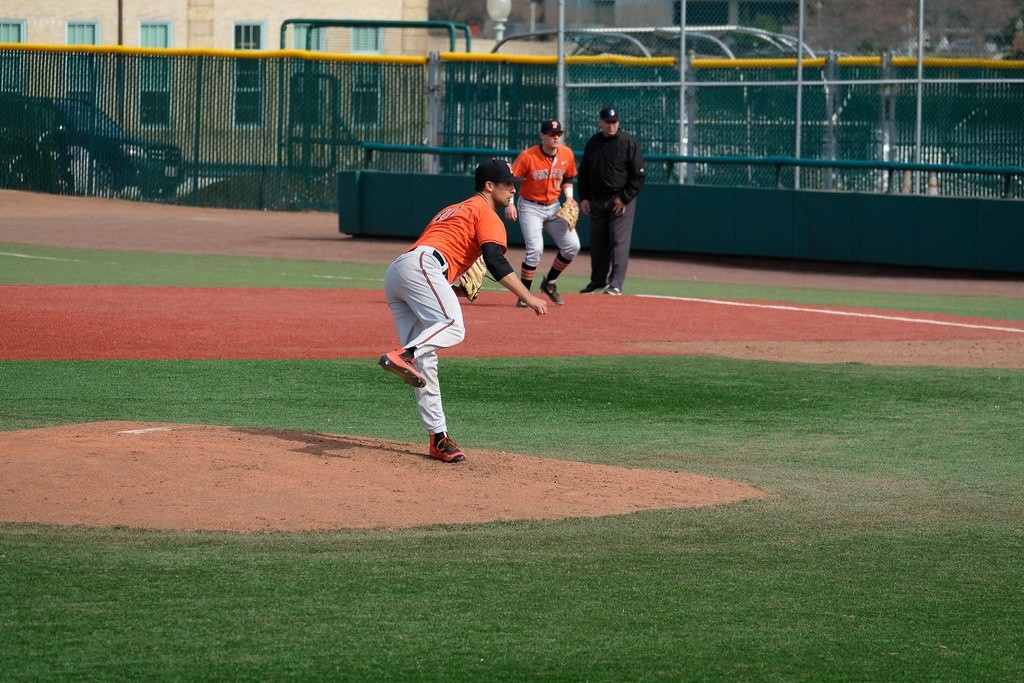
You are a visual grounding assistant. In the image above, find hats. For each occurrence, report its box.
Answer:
[475,158,527,182]
[540,119,565,133]
[600,107,621,122]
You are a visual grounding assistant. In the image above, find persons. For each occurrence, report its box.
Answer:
[577,106,646,296]
[503,120,581,309]
[380,157,549,464]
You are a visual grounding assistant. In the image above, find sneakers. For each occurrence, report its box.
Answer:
[540,277,564,305]
[580,281,610,294]
[604,285,622,295]
[429,432,467,462]
[377,349,427,388]
[517,299,528,308]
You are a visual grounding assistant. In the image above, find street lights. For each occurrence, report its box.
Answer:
[486,0,511,113]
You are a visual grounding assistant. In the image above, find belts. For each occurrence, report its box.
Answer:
[411,247,447,279]
[523,198,550,206]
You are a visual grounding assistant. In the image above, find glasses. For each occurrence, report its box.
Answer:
[605,120,618,124]
[547,132,562,137]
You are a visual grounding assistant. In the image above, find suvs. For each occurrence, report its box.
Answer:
[0,94,188,200]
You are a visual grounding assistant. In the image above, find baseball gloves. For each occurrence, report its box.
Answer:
[555,197,580,233]
[458,254,487,302]
